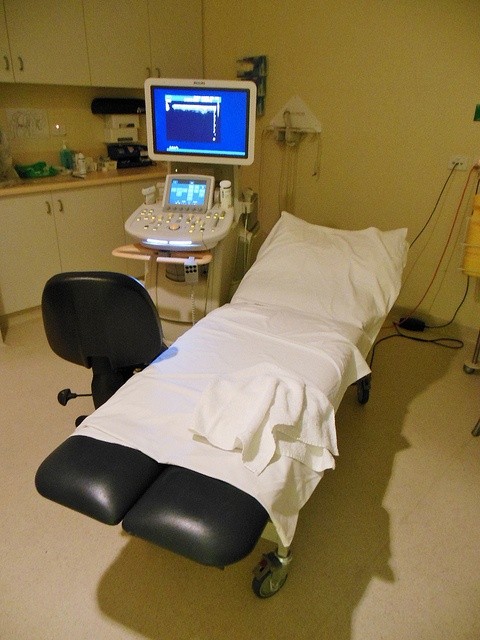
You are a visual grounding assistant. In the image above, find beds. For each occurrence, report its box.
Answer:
[34,212,410,600]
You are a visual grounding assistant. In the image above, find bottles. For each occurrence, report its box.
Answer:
[76,154,88,176]
[60,139,74,175]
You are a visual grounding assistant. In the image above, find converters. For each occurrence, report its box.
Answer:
[398,316,428,333]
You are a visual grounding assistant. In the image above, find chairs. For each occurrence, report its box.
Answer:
[42,272,168,424]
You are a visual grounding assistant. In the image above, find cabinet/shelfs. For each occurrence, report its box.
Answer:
[84,1,204,88]
[122,178,167,277]
[0,0,91,87]
[0,182,128,321]
